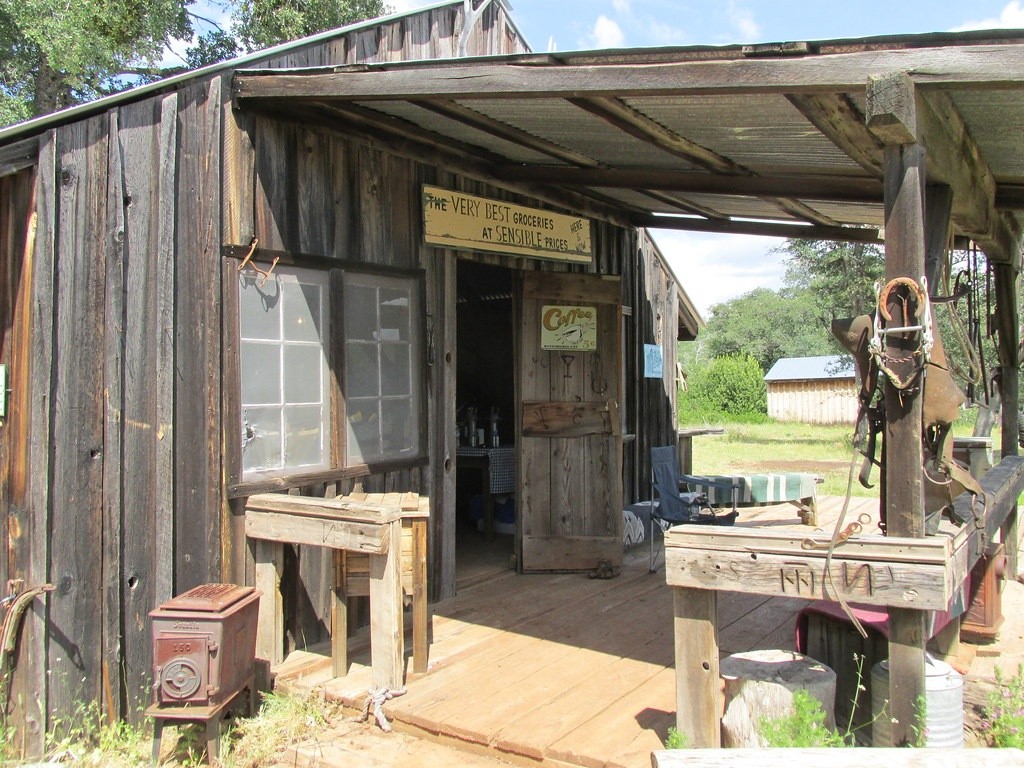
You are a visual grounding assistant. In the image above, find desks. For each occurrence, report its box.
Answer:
[456,444,515,543]
[332,491,431,675]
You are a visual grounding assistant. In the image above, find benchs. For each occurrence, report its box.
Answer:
[622,492,706,552]
[679,472,825,526]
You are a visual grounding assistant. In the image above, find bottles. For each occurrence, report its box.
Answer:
[456,425,460,447]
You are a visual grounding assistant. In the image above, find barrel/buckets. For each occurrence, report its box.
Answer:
[869,648,964,748]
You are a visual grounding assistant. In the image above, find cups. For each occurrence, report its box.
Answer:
[477,428,488,444]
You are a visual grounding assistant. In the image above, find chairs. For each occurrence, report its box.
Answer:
[650,445,739,574]
